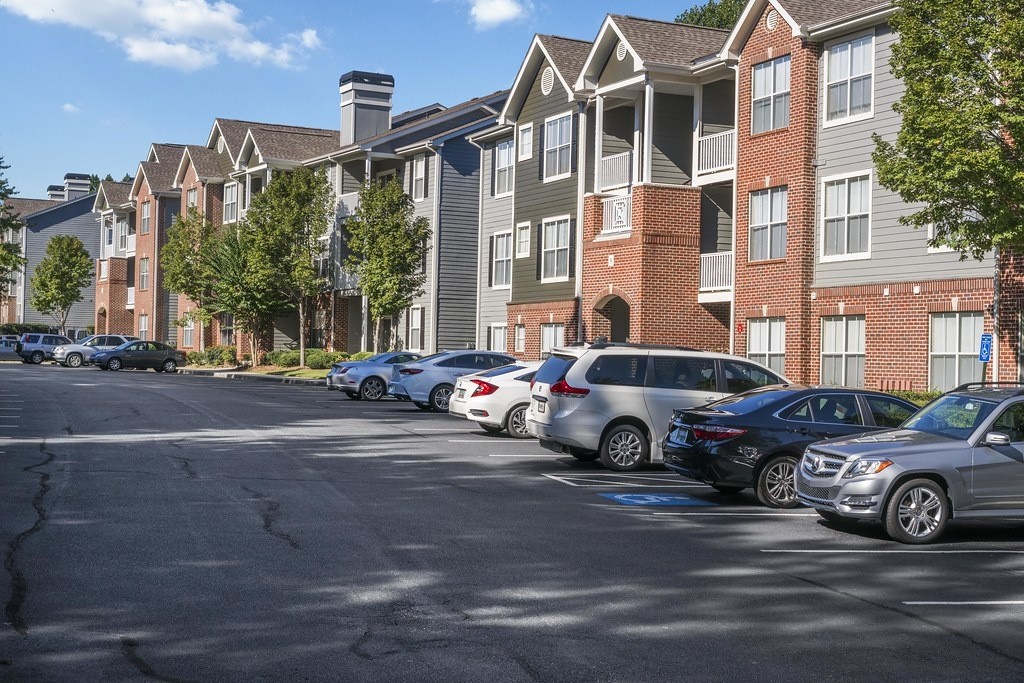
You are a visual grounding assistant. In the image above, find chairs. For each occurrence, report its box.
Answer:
[998,410,1019,442]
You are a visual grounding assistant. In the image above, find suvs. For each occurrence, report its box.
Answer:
[51,334,147,369]
[13,332,75,365]
[792,381,1024,545]
[523,335,846,473]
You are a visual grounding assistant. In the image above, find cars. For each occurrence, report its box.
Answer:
[388,349,522,414]
[87,339,188,374]
[662,382,963,510]
[325,350,424,402]
[447,359,548,438]
[0,334,27,363]
